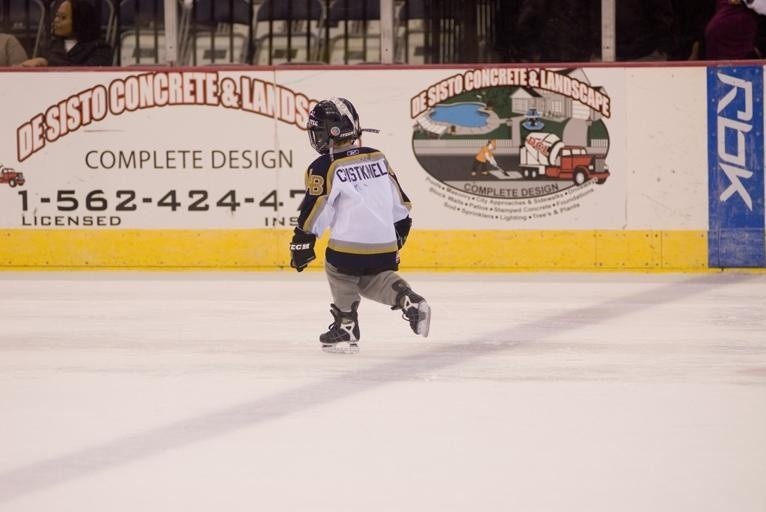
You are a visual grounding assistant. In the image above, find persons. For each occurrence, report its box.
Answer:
[568,0,766,62]
[451,10,521,62]
[0,0,112,66]
[287,98,433,355]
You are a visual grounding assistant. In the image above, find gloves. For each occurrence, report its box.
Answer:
[290,228,317,272]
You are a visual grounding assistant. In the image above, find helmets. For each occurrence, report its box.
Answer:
[308,97,361,154]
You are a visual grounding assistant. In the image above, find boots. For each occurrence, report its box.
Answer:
[319,312,360,343]
[396,288,426,334]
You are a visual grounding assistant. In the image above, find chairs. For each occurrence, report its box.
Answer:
[80,1,476,67]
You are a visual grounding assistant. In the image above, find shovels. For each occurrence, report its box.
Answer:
[497,166,509,176]
